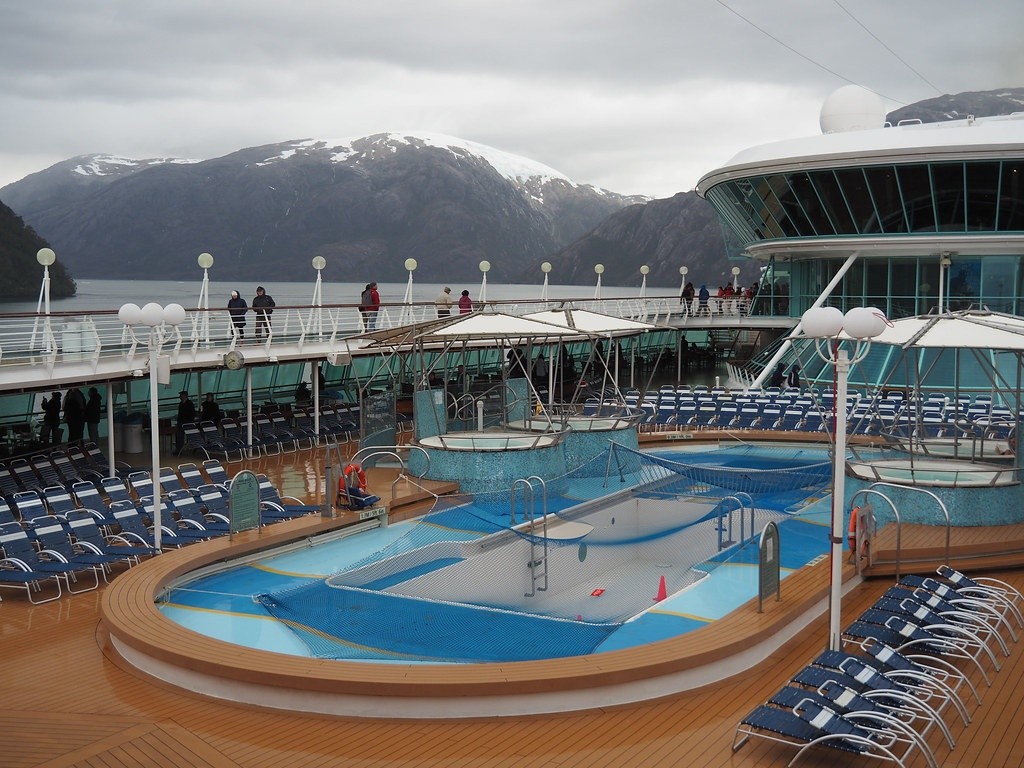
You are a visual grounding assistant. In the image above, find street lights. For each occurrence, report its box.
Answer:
[198,252,214,350]
[479,260,491,303]
[404,258,418,325]
[640,264,650,316]
[311,255,327,343]
[731,266,740,315]
[798,305,887,654]
[541,261,552,311]
[36,247,56,354]
[117,301,186,555]
[594,263,604,313]
[679,266,688,304]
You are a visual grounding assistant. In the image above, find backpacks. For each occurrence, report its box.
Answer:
[362,290,379,306]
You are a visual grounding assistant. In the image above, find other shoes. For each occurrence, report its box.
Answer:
[239,342,244,347]
[253,342,261,346]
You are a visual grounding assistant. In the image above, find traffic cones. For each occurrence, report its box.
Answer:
[653,575,667,602]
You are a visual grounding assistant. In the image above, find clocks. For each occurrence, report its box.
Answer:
[226,352,244,370]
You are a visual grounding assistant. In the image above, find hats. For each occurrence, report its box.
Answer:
[232,291,237,296]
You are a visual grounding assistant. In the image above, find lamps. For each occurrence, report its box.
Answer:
[131,370,143,376]
[326,352,351,367]
[940,252,951,268]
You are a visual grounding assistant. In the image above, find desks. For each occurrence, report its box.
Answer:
[3,434,40,440]
[151,427,179,453]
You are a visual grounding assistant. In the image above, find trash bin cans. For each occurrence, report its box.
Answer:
[113,410,145,454]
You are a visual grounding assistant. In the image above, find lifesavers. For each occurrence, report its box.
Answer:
[340,463,368,500]
[848,506,867,558]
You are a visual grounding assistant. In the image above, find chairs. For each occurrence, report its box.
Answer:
[582,385,1024,441]
[0,441,328,605]
[732,565,1024,768]
[145,373,567,464]
[0,424,64,458]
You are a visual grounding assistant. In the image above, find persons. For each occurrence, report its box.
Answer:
[715,281,757,317]
[760,282,789,315]
[435,287,452,319]
[706,333,716,351]
[251,286,275,343]
[694,285,709,316]
[535,355,549,383]
[361,283,380,332]
[318,366,326,407]
[169,390,196,456]
[679,282,695,317]
[199,392,220,429]
[227,290,248,347]
[681,335,689,369]
[771,362,801,388]
[295,380,311,401]
[558,344,576,368]
[506,346,526,379]
[612,340,625,367]
[593,341,606,363]
[62,387,87,441]
[83,385,102,445]
[995,437,1015,455]
[459,290,474,315]
[40,392,61,444]
